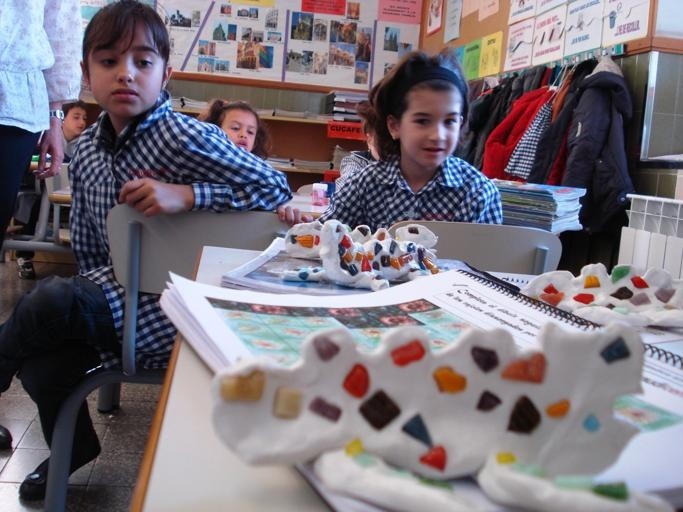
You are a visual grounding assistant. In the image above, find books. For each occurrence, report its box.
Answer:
[489,175,587,234]
[260,144,350,171]
[172,90,370,122]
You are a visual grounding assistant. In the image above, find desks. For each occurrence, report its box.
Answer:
[48,190,73,205]
[129,245,682,512]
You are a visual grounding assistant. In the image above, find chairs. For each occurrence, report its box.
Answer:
[387,220,562,276]
[24,203,334,512]
[2,160,79,252]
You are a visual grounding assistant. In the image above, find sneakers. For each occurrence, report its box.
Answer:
[19,432,101,500]
[16,257,35,280]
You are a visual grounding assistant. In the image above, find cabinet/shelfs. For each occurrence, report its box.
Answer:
[178,109,367,176]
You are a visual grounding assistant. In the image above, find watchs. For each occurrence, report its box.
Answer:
[48,110,65,121]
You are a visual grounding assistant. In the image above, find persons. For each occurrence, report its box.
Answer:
[0,1,294,504]
[200,96,272,161]
[11,98,87,281]
[285,0,441,84]
[334,99,380,192]
[1,0,86,250]
[276,46,504,232]
